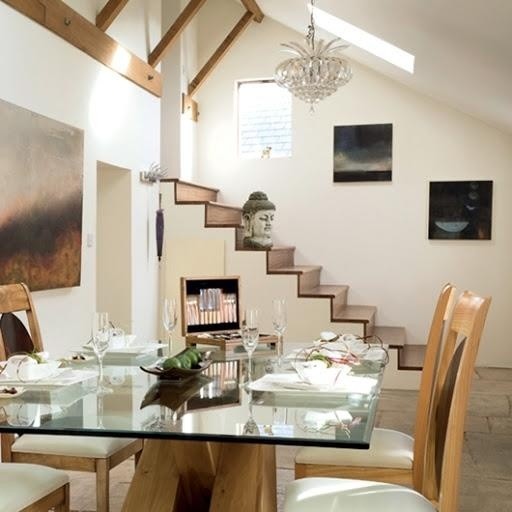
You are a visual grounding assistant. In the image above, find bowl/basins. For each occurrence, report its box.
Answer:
[108,334,138,350]
[0,354,64,381]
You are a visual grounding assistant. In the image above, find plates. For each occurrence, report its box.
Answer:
[73,360,161,376]
[0,383,98,407]
[1,386,29,398]
[238,373,379,397]
[244,391,377,411]
[284,347,390,363]
[139,348,214,376]
[70,342,169,360]
[140,374,215,410]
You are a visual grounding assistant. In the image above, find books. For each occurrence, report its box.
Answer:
[189,292,236,326]
[200,361,237,399]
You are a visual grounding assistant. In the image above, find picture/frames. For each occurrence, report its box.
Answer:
[333,124,394,182]
[428,179,494,241]
[1,98,83,290]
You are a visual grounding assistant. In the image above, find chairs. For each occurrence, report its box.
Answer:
[280,291,490,512]
[0,462,71,511]
[294,283,455,492]
[0,284,141,512]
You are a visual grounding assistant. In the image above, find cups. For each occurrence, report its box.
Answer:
[240,309,260,392]
[271,296,288,363]
[161,296,178,356]
[91,311,114,394]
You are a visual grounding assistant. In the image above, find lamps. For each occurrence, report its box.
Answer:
[273,0,355,111]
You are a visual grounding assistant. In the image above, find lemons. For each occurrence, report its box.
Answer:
[163,358,181,370]
[189,348,202,362]
[177,355,191,369]
[184,351,197,365]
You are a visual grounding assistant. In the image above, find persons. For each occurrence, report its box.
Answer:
[241,190,275,247]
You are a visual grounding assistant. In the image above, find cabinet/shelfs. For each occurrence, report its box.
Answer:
[182,275,245,334]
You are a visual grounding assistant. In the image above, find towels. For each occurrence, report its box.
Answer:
[247,371,379,396]
[288,341,389,362]
[71,338,167,354]
